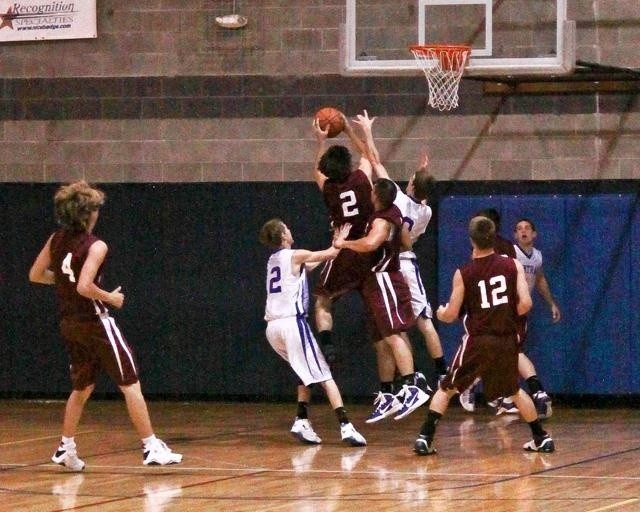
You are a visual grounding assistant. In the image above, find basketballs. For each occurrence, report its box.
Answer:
[316,108,344,138]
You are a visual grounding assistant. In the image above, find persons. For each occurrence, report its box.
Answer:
[350,108,449,378]
[470,207,554,420]
[412,214,556,457]
[28,179,183,472]
[330,178,432,425]
[457,217,562,415]
[256,217,367,447]
[309,110,375,373]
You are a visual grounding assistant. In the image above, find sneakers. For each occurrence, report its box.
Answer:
[142,439,183,466]
[290,418,322,444]
[340,422,367,446]
[365,367,553,424]
[522,432,554,453]
[414,433,436,454]
[51,443,85,472]
